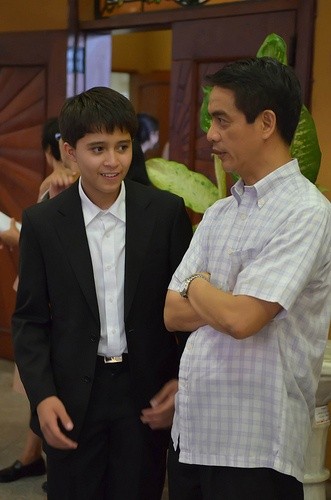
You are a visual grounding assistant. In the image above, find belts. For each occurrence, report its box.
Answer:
[96,353,129,366]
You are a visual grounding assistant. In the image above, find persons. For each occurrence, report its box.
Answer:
[0,86,193,500]
[164,55,331,500]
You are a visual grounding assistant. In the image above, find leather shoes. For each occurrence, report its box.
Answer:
[42,482,48,491]
[0,457,47,482]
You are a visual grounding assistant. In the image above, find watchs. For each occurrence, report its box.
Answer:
[179,274,210,298]
